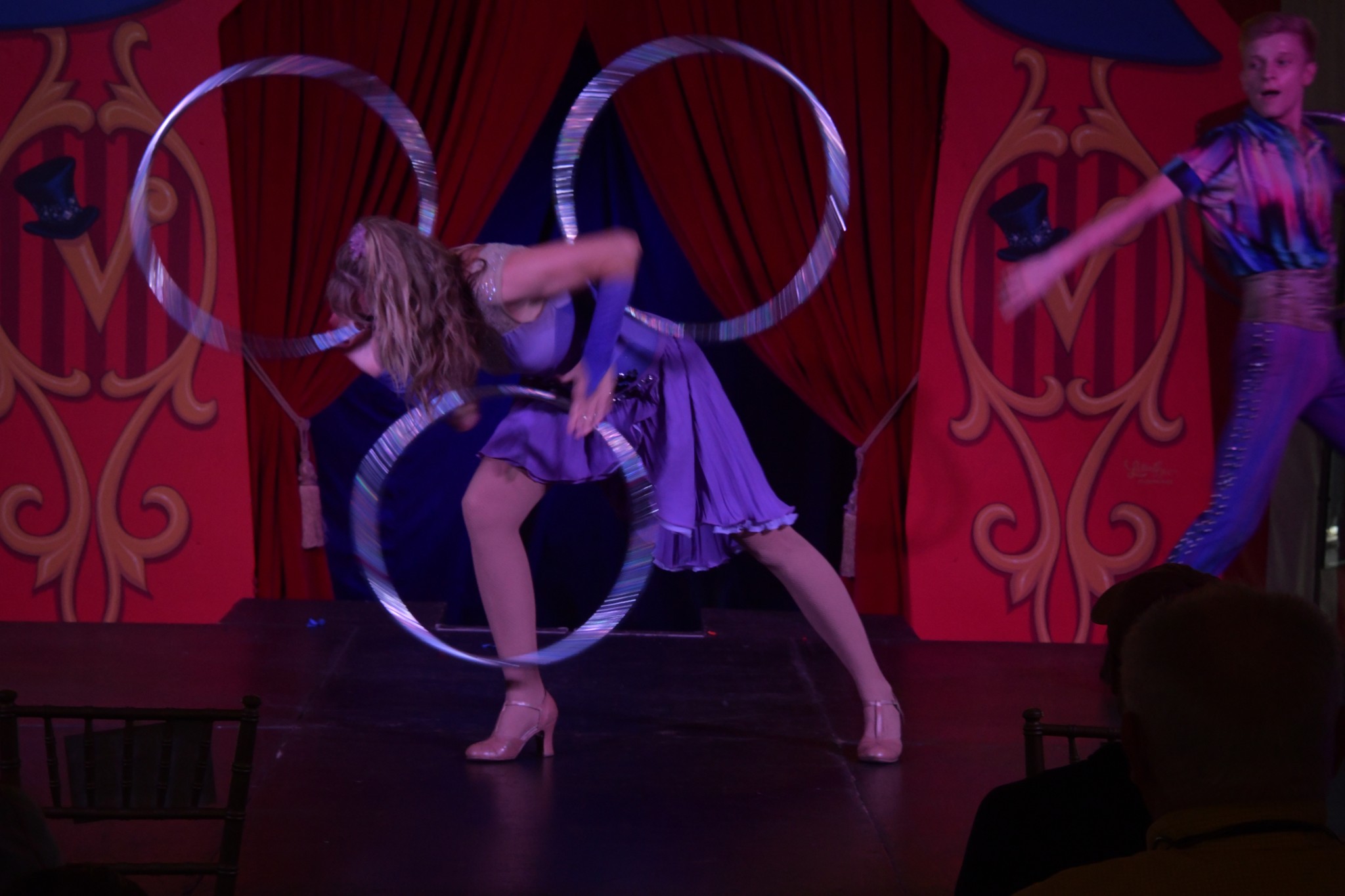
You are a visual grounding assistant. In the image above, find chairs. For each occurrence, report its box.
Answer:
[0,687,262,896]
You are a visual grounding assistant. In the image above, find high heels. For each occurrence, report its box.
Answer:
[464,690,558,760]
[857,696,904,762]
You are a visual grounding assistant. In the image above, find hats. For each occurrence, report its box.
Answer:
[1091,562,1220,646]
[988,183,1070,261]
[15,158,99,238]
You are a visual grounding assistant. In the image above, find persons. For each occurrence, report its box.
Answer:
[322,218,905,763]
[1000,16,1345,576]
[953,563,1345,896]
